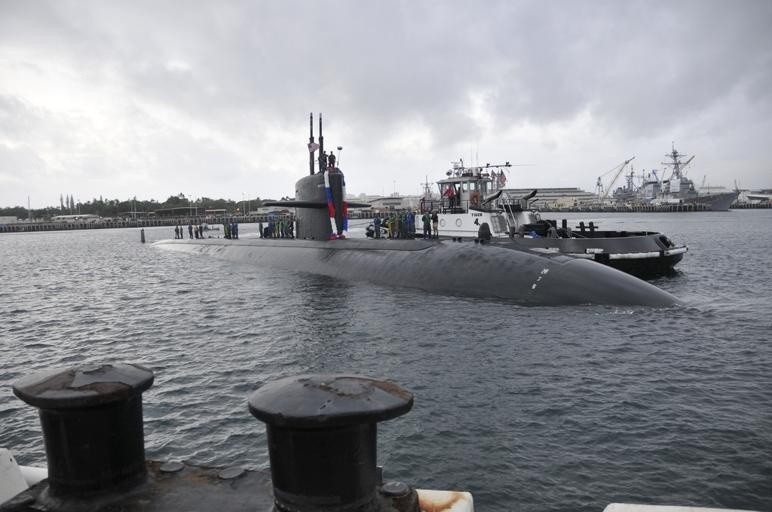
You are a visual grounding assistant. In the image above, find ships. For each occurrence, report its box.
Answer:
[597,140,742,211]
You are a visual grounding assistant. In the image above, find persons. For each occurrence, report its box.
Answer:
[223,219,238,239]
[188,223,202,239]
[373,208,416,240]
[258,215,294,239]
[422,211,439,240]
[175,225,183,239]
[321,151,335,169]
[443,186,455,208]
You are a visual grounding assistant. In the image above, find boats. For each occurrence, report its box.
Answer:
[746,189,772,200]
[367,159,691,277]
